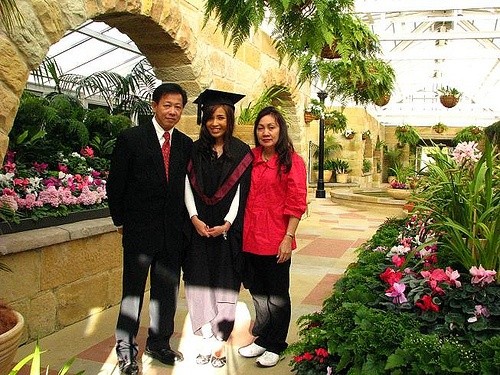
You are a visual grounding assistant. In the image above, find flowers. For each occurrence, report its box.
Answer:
[0,147,111,224]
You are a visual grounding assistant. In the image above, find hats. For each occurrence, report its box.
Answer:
[193,89,246,125]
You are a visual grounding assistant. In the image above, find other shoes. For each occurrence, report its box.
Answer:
[196,345,210,364]
[211,342,226,367]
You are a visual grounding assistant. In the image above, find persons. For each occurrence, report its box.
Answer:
[239,105,308,368]
[183,89,255,368]
[106,82,193,375]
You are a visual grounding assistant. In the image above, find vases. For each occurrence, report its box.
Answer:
[0,306,25,375]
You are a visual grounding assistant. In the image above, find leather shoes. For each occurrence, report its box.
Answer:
[145,345,183,366]
[119,359,139,375]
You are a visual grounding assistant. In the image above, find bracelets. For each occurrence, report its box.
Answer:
[285,232,295,240]
[222,225,227,240]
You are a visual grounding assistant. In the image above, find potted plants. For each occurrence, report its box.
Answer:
[334,158,349,183]
[396,124,419,146]
[455,125,483,141]
[433,123,447,133]
[310,140,342,183]
[385,150,404,185]
[438,86,461,108]
[304,15,394,139]
[0,206,112,235]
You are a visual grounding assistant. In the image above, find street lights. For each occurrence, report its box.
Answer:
[316,89,328,198]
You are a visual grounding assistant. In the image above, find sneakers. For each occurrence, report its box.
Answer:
[238,342,264,357]
[256,351,280,367]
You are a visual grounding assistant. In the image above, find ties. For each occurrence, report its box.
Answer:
[161,132,170,180]
